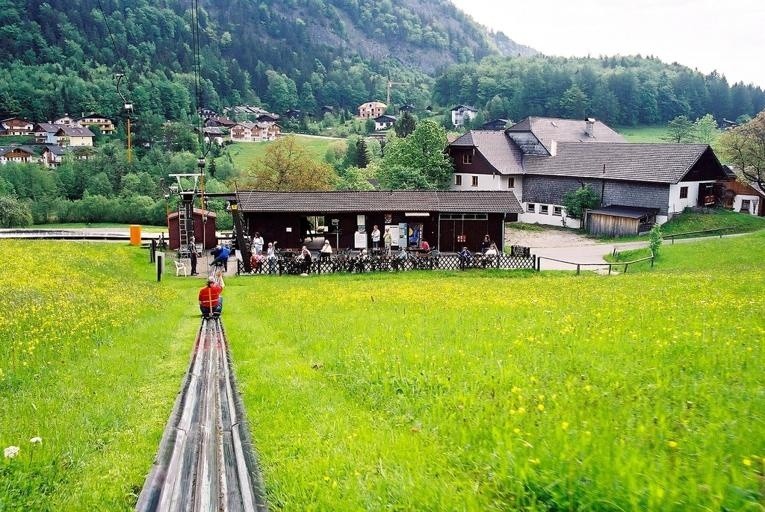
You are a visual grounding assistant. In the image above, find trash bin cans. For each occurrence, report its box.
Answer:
[391,244,399,257]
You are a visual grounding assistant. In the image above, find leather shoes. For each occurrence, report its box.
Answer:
[191,271,199,276]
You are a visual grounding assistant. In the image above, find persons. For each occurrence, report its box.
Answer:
[394,247,407,260]
[420,238,430,252]
[481,234,491,254]
[298,244,311,260]
[210,245,221,259]
[481,244,497,268]
[370,224,380,248]
[209,244,230,272]
[199,271,225,320]
[320,239,332,253]
[457,244,473,267]
[187,237,199,276]
[266,241,277,257]
[301,249,311,271]
[383,228,393,257]
[252,231,264,255]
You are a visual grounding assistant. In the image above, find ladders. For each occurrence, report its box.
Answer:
[179,204,188,247]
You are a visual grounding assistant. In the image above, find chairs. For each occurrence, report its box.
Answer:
[320,252,330,261]
[175,261,186,277]
[214,255,227,272]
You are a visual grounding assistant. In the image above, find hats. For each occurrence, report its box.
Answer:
[207,276,216,283]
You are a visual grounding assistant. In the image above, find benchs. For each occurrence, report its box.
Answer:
[180,243,202,257]
